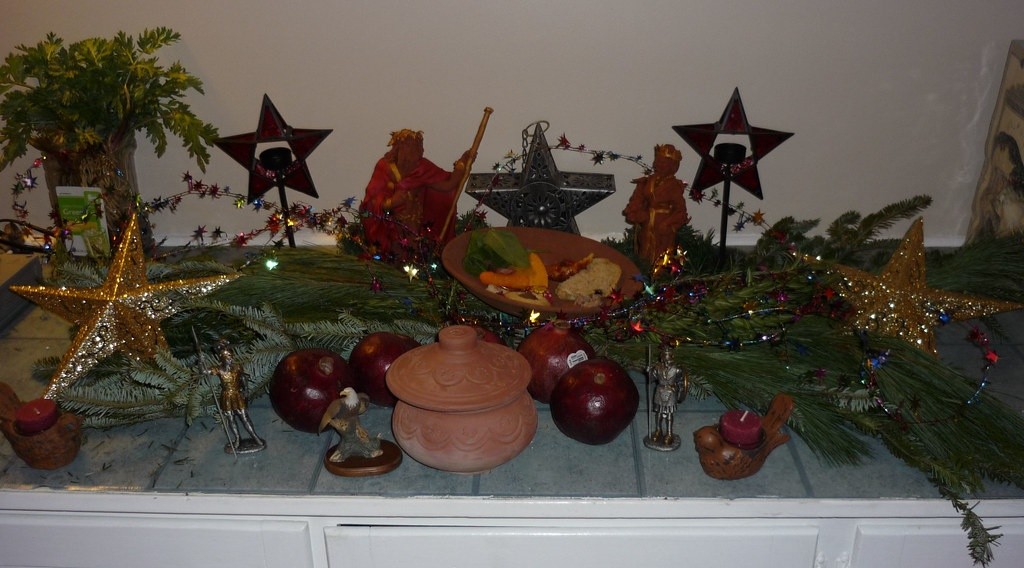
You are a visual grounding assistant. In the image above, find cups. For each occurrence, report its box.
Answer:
[385,327,538,473]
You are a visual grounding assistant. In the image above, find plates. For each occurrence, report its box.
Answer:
[442,225,642,323]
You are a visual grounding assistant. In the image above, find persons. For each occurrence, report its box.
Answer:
[621,142,687,266]
[359,128,478,259]
[646,345,687,444]
[198,339,264,447]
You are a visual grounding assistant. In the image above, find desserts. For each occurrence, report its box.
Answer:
[479,253,549,289]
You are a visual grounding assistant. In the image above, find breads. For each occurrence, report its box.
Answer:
[557,258,622,304]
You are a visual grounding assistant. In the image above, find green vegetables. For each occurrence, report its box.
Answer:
[465,228,531,274]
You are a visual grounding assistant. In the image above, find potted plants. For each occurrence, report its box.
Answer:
[0,26,220,252]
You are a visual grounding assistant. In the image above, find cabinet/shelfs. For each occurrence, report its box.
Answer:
[0,511,1024,568]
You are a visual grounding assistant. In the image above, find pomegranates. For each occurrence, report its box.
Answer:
[270,322,639,446]
